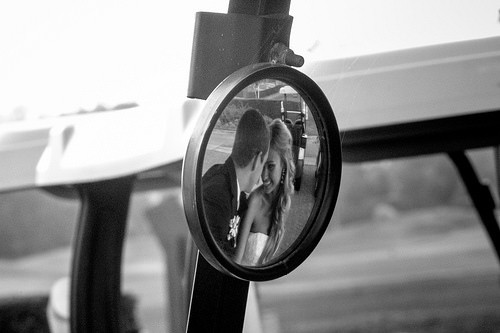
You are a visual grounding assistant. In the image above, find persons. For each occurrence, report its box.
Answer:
[200,109,270,262]
[233,116,297,266]
